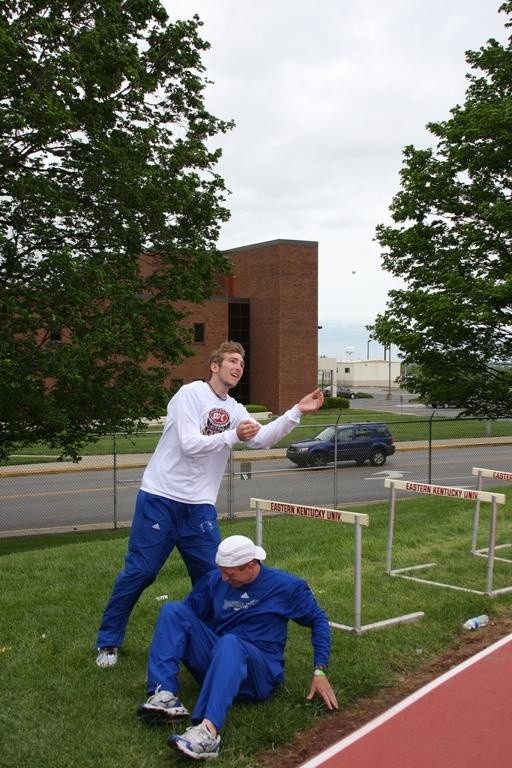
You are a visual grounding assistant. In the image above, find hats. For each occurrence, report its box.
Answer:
[213,533,267,568]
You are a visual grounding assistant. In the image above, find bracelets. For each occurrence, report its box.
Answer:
[313,669,326,676]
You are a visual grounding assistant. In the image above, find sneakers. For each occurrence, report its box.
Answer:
[136,689,191,725]
[168,724,222,760]
[95,645,118,667]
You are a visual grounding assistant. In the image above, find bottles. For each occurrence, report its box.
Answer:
[461,614,490,630]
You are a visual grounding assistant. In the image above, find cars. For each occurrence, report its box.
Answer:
[323,384,357,399]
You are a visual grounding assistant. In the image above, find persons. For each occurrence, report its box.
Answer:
[93,338,325,670]
[135,535,339,759]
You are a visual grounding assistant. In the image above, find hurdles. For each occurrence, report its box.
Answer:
[384,478,512,598]
[471,467,512,566]
[250,497,425,634]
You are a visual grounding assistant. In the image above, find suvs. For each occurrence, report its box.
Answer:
[287,421,396,470]
